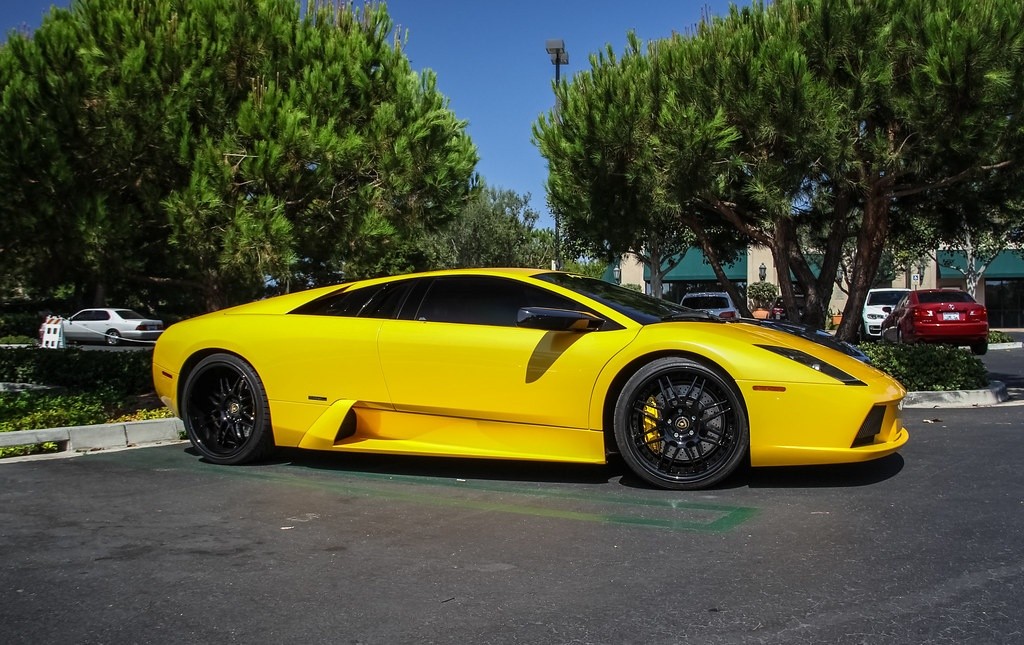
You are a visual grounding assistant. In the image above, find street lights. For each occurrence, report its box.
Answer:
[546,39,571,269]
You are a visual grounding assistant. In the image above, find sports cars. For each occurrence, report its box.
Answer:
[151,267,911,492]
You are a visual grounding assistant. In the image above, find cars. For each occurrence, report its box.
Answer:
[880,288,990,357]
[39,308,165,346]
[678,291,742,319]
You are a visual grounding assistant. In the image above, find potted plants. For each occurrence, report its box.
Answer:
[747,280,779,319]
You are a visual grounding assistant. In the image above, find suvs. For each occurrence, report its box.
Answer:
[768,294,805,324]
[860,287,912,343]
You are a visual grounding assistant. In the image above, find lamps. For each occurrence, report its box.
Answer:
[917,262,924,280]
[613,265,621,285]
[759,262,766,280]
